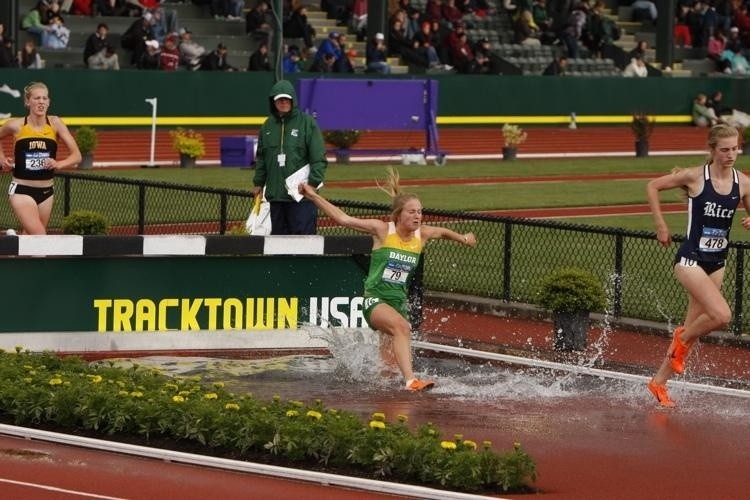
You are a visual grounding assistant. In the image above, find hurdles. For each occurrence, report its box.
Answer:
[0,234,424,355]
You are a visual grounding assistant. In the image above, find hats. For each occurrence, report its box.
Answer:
[273,93,293,101]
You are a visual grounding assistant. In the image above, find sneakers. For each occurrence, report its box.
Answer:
[6,229,16,258]
[646,378,676,408]
[406,378,436,390]
[666,326,692,376]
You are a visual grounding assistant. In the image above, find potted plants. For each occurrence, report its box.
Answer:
[631,109,658,158]
[323,128,364,164]
[535,264,614,356]
[499,123,527,160]
[169,127,206,168]
[73,126,98,172]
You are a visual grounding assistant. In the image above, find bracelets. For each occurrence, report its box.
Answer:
[464,235,467,245]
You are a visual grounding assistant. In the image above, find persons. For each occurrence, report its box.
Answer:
[253,79,328,236]
[1,1,750,78]
[298,166,477,393]
[707,91,736,118]
[646,124,750,408]
[692,93,721,128]
[0,81,82,236]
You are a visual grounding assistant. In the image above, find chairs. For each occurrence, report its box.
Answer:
[0,0,750,79]
